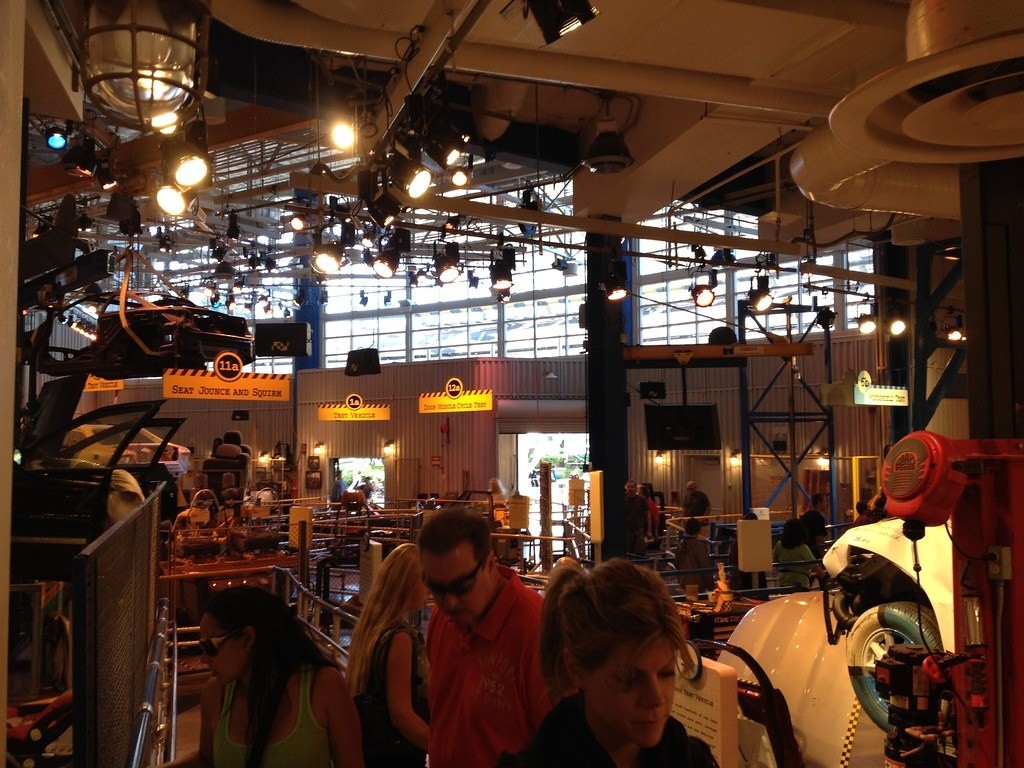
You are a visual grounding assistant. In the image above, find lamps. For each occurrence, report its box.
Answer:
[815,448,828,465]
[24,0,968,344]
[729,449,740,466]
[383,439,395,454]
[655,450,663,464]
[314,440,325,453]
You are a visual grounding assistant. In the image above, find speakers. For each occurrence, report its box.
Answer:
[255,323,312,357]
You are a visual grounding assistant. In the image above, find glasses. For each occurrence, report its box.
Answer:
[421,557,485,596]
[198,626,243,657]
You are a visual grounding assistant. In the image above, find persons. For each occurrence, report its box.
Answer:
[344,544,432,767]
[771,518,827,594]
[417,508,552,768]
[497,556,721,768]
[488,478,506,501]
[682,480,712,527]
[331,469,349,509]
[675,517,715,601]
[623,478,659,561]
[798,493,828,594]
[164,491,211,557]
[147,586,367,768]
[727,513,768,595]
[352,478,375,503]
[852,495,886,527]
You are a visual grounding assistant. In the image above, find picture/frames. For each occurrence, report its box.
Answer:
[306,470,322,489]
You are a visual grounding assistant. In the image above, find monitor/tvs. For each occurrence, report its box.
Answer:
[644,402,721,450]
